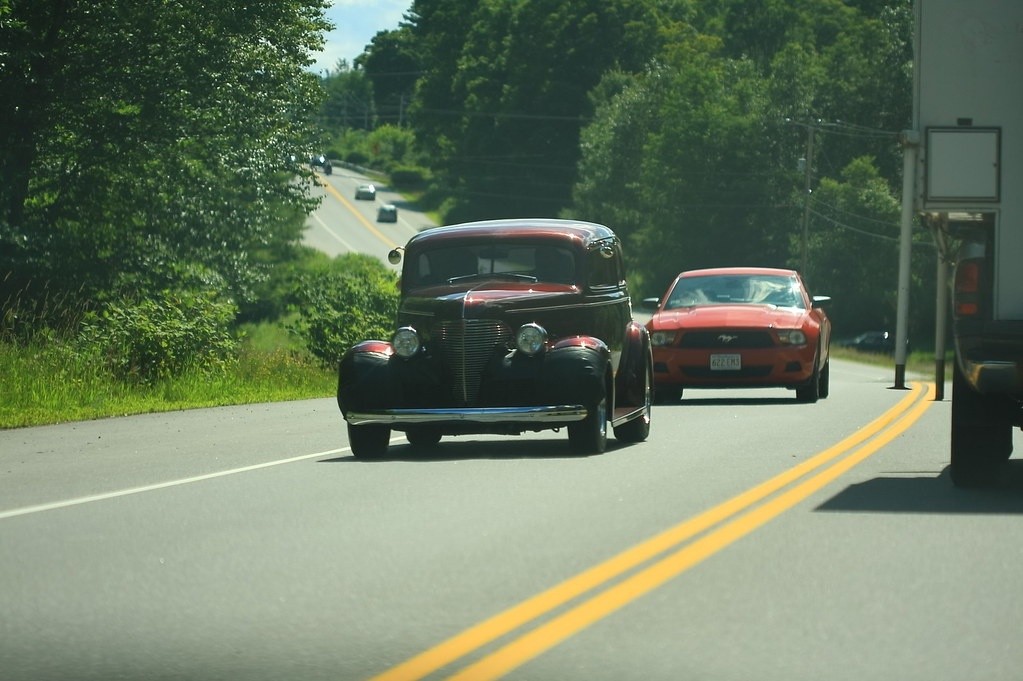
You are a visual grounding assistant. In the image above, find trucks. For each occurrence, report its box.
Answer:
[912,0,1023,486]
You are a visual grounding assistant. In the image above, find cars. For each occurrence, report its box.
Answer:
[311,154,326,166]
[337,219,653,457]
[377,205,396,223]
[355,184,376,200]
[644,268,831,406]
[842,332,912,357]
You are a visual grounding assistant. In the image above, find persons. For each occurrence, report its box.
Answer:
[527,247,571,282]
